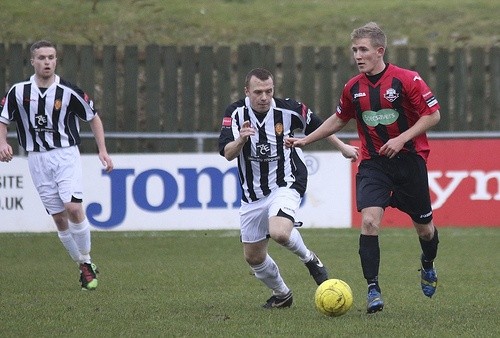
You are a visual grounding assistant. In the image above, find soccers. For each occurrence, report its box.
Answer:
[314,279,353,317]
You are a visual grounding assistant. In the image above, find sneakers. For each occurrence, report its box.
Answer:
[79,262,99,291]
[304,251,328,286]
[262,291,293,309]
[366,285,383,313]
[420,254,437,297]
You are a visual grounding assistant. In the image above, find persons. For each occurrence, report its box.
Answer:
[0,39,114,291]
[287,22,442,312]
[219,67,361,312]
